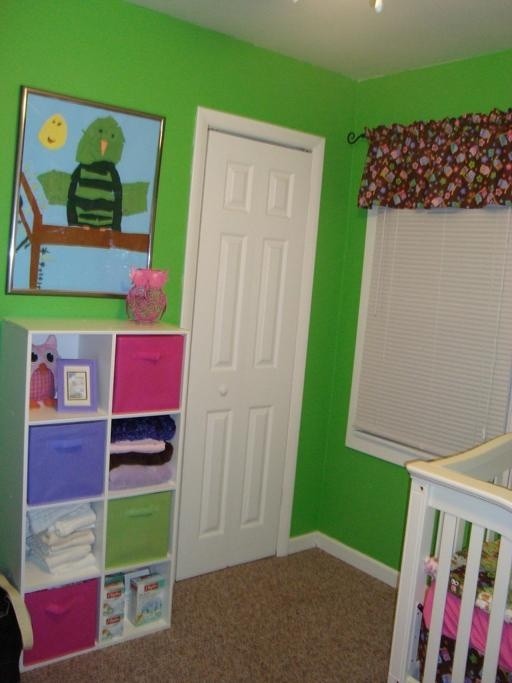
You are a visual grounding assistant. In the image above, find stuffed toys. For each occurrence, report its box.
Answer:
[28,334,62,409]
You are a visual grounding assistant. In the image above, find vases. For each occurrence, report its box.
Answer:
[128,267,169,320]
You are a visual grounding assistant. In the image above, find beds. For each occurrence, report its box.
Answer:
[376,433,511,683]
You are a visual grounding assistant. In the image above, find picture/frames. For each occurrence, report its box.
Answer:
[1,82,168,299]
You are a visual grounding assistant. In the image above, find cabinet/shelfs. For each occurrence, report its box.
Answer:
[6,318,192,672]
